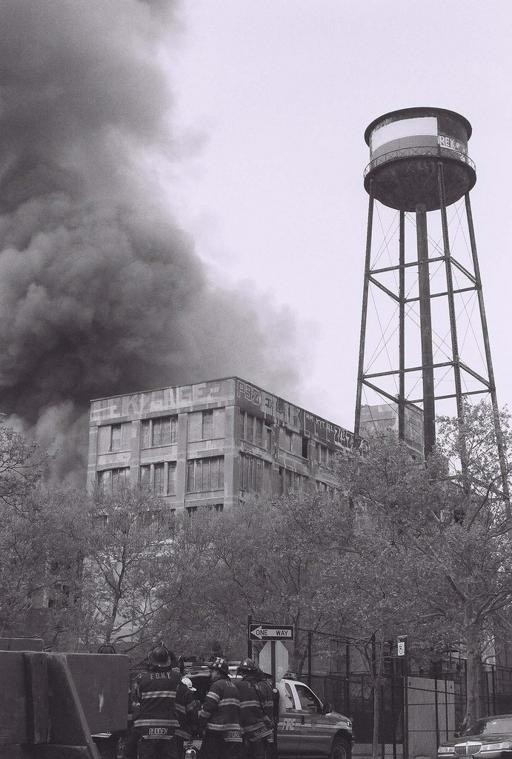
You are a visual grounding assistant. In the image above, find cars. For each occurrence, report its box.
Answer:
[437,714,512,759]
[183,661,354,759]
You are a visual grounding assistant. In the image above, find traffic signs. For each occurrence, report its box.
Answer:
[248,620,295,646]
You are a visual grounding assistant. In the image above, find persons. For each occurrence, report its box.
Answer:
[235,657,275,759]
[197,655,243,759]
[132,644,197,758]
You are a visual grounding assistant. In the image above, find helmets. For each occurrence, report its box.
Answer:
[237,658,259,671]
[147,646,170,668]
[208,657,232,676]
